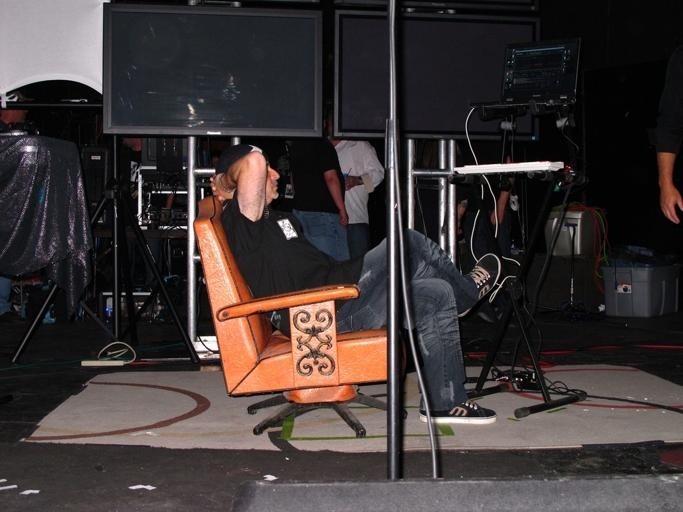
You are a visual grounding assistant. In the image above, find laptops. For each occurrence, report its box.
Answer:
[468,34,581,113]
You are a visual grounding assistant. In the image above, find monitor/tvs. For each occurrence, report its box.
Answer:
[102,2,323,137]
[333,9,541,141]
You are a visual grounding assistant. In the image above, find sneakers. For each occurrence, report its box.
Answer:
[419,399,497,425]
[457,253,501,318]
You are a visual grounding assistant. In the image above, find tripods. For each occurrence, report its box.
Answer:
[12,137,199,367]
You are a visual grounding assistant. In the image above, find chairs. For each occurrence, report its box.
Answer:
[192,196,408,441]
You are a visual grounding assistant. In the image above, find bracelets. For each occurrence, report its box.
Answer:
[218,173,235,194]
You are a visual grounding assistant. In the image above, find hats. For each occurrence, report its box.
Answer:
[215,144,270,176]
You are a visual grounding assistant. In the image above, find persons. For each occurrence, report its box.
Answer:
[143,192,175,286]
[325,111,385,267]
[208,144,502,426]
[1,91,32,327]
[427,139,528,277]
[654,47,682,225]
[290,132,352,263]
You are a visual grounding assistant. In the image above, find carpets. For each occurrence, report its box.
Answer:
[19,361,683,454]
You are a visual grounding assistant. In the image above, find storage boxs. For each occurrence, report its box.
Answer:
[601,263,682,318]
